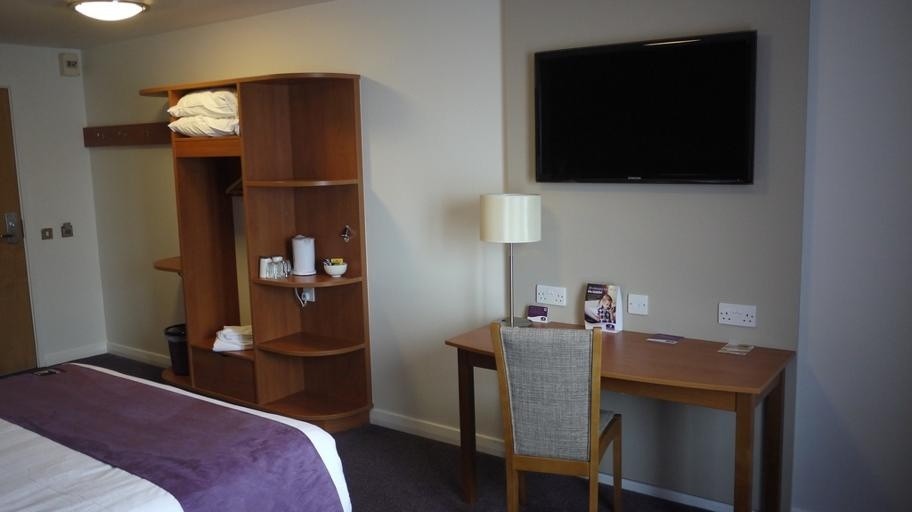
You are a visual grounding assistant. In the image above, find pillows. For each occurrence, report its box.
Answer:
[167,114,239,137]
[168,90,239,118]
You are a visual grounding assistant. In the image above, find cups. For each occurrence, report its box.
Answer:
[259,254,292,279]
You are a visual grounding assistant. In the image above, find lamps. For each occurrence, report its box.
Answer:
[479,194,550,329]
[67,0,153,23]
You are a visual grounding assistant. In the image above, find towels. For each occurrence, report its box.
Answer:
[213,325,253,353]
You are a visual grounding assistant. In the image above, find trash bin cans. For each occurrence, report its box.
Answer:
[164,324,189,376]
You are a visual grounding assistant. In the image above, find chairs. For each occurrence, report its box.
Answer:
[490,323,623,512]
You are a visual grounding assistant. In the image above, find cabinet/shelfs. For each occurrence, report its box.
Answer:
[138,72,372,432]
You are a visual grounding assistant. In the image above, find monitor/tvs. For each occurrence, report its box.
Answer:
[533,30,758,189]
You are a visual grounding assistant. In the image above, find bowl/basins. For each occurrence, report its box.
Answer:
[324,263,347,278]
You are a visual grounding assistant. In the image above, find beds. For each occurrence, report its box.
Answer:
[0,362,353,512]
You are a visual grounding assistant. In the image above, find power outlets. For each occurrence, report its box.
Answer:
[718,303,756,327]
[536,284,566,306]
[627,294,648,315]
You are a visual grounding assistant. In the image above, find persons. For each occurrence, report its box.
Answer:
[592,295,615,328]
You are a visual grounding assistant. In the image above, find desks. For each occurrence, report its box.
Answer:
[445,318,797,512]
